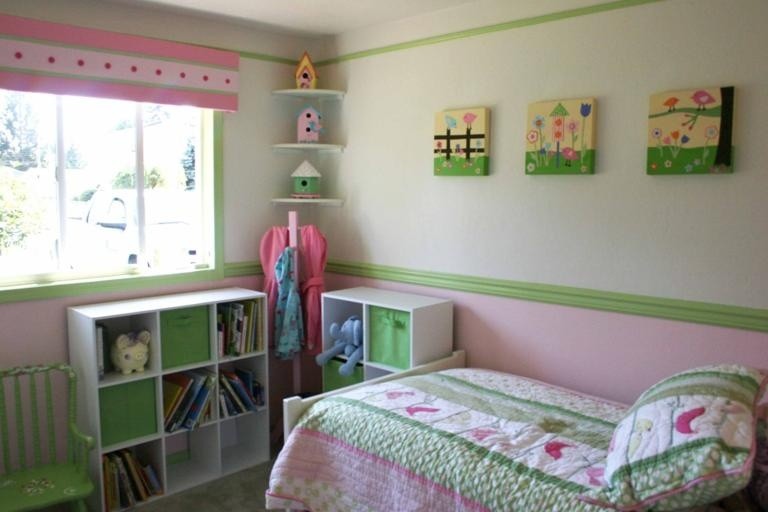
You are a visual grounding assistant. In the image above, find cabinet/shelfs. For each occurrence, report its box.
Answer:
[320,287,455,394]
[271,86,345,207]
[69,285,271,510]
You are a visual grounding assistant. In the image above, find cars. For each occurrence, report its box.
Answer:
[69,186,197,265]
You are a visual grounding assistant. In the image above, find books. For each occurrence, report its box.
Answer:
[220,365,264,419]
[96,327,103,375]
[163,369,218,433]
[217,299,263,360]
[104,451,164,512]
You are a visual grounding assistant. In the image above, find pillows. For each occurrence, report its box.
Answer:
[576,363,767,511]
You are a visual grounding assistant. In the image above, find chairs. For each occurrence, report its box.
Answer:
[0,363,98,511]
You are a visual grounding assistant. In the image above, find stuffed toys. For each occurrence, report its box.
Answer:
[316,314,363,376]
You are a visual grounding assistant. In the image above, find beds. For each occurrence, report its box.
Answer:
[282,349,767,512]
